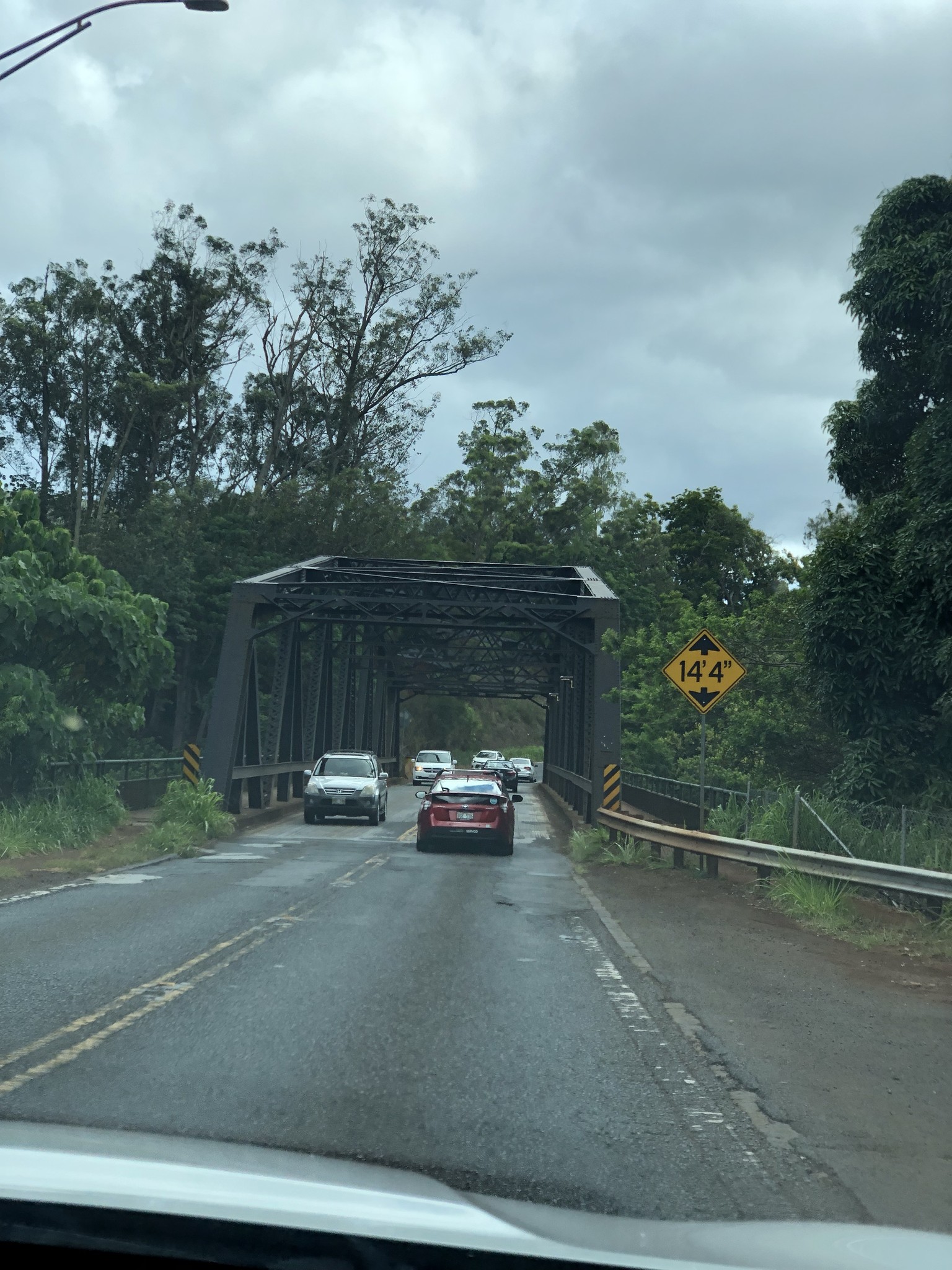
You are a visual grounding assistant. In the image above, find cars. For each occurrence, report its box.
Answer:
[414,768,524,856]
[471,750,505,770]
[509,758,538,783]
[477,760,521,793]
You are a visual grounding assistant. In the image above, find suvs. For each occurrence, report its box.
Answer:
[411,750,457,786]
[303,749,388,825]
